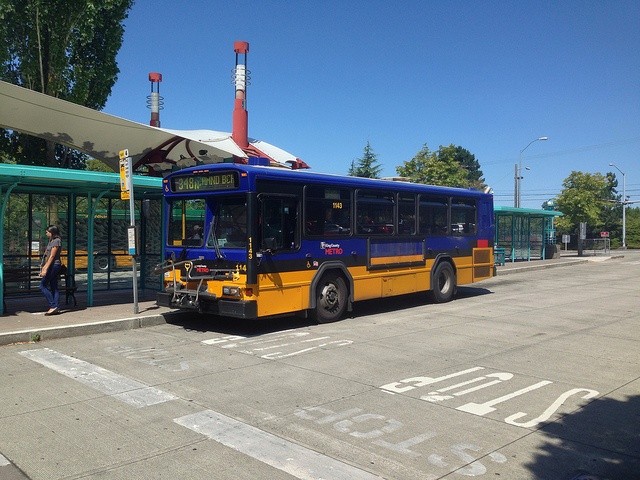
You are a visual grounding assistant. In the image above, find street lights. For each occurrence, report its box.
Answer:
[513,164,531,208]
[608,163,626,247]
[518,136,548,208]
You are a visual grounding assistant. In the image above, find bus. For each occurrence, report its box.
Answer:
[154,164,494,323]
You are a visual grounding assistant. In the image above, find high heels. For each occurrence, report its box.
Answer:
[45,307,60,316]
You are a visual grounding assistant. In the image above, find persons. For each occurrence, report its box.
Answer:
[39,225,62,316]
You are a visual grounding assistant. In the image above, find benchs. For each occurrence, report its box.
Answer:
[3,264,81,313]
